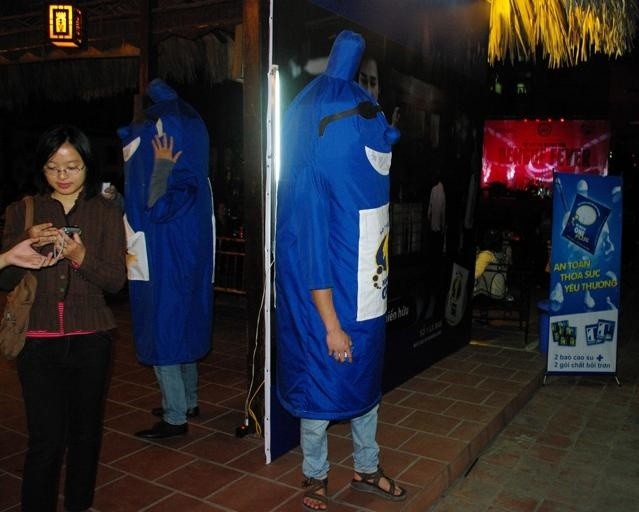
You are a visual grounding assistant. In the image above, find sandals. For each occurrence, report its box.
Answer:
[351,463,409,502]
[299,472,329,512]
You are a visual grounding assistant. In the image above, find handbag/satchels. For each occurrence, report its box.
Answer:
[1,195,41,363]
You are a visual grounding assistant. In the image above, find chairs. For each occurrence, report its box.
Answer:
[472,259,537,346]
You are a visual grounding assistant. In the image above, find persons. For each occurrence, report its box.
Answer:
[0,236,65,273]
[0,125,125,510]
[274,27,406,510]
[213,197,237,272]
[103,64,213,443]
[419,166,447,319]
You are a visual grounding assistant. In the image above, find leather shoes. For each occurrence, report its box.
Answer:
[151,404,210,420]
[134,419,190,439]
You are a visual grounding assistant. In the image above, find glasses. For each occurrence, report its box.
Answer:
[43,163,85,177]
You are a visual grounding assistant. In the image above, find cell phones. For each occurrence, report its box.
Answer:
[60,225,82,234]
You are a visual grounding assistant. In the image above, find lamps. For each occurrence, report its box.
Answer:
[47,1,83,49]
